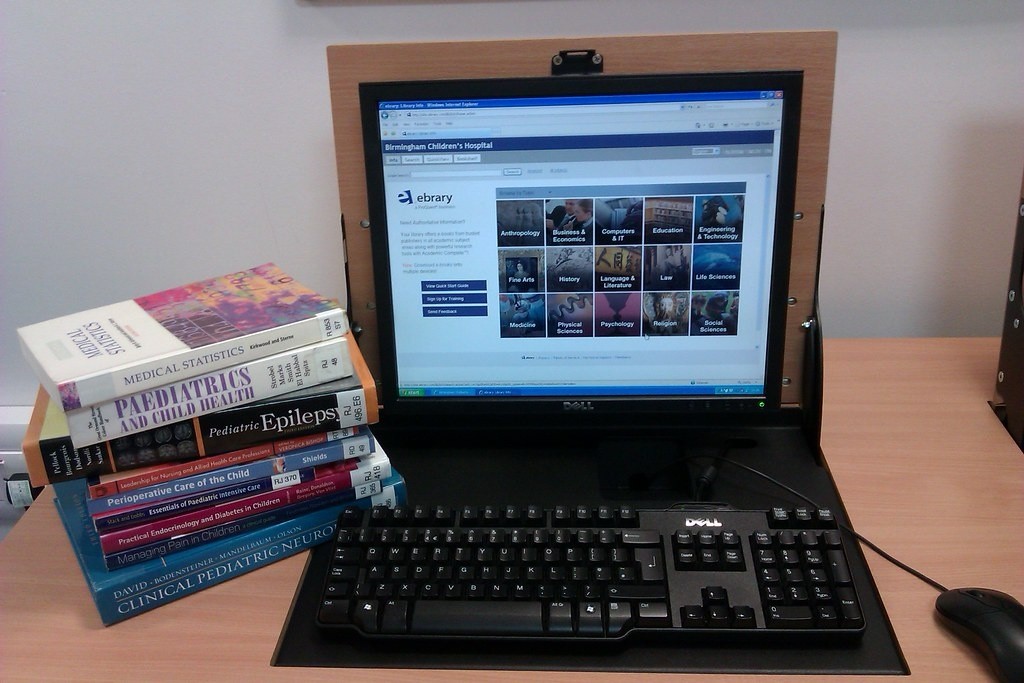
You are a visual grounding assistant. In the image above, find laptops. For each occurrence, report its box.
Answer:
[276,70,904,674]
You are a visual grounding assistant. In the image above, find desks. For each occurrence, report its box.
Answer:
[0,336,1024,683]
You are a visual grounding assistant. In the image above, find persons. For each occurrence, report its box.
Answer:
[514,262,529,278]
[546,198,593,245]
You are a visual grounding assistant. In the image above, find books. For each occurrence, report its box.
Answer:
[17,262,407,627]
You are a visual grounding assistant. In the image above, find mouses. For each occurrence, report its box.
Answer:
[935,588,1024,683]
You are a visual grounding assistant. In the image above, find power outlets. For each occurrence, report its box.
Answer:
[0,451,28,501]
